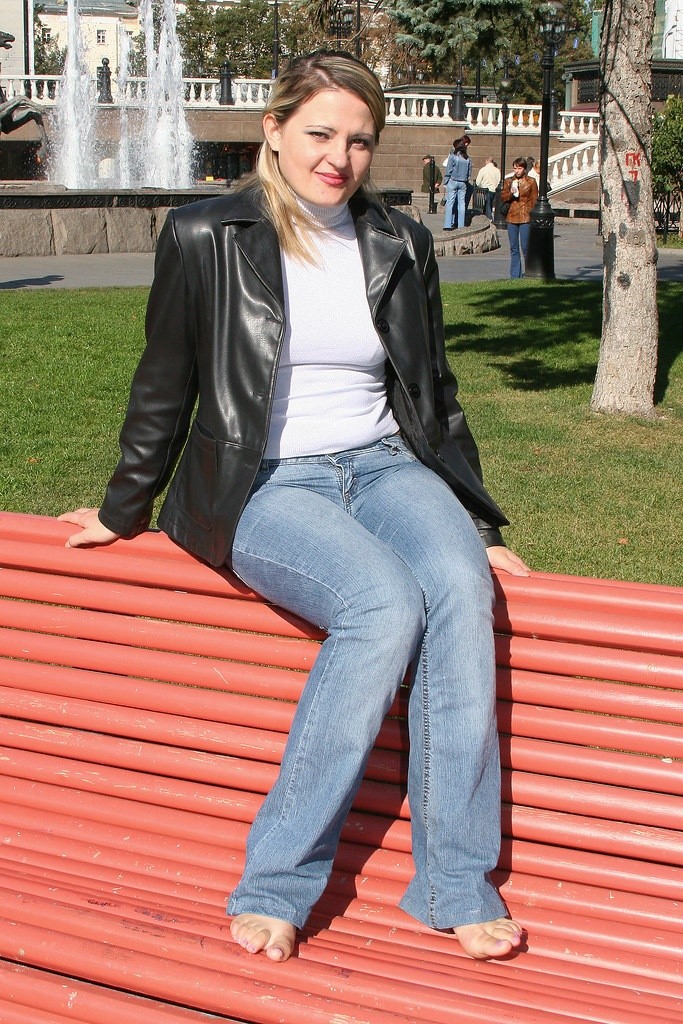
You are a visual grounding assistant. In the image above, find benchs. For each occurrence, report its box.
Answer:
[3,512,683,1024]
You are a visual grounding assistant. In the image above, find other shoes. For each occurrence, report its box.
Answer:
[443,227,452,231]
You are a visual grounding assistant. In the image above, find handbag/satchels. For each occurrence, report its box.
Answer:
[500,201,511,216]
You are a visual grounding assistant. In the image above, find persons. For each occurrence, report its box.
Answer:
[501,157,552,281]
[443,134,473,230]
[420,155,442,214]
[57,50,531,963]
[476,156,501,222]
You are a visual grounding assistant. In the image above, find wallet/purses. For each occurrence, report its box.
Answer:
[440,197,447,206]
